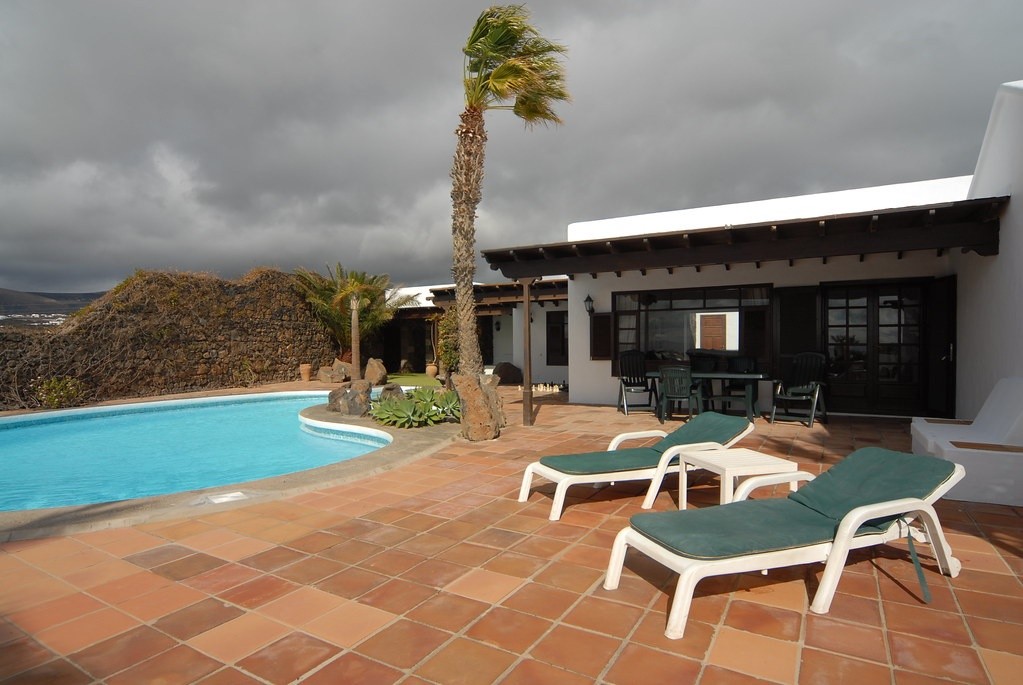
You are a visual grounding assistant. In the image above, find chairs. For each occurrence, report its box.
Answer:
[602,446,967,639]
[517,412,756,520]
[686,348,720,411]
[618,349,658,417]
[659,365,693,424]
[722,354,759,409]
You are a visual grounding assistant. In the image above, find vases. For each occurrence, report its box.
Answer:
[299,363,312,381]
[426,364,438,378]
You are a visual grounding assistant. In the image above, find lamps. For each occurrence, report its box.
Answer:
[495,321,500,331]
[584,294,595,316]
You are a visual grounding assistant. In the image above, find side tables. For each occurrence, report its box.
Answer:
[641,440,818,575]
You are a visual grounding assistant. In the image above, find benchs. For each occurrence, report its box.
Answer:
[911,378,1023,508]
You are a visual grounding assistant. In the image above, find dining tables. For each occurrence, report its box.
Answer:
[644,371,769,425]
[770,351,828,429]
